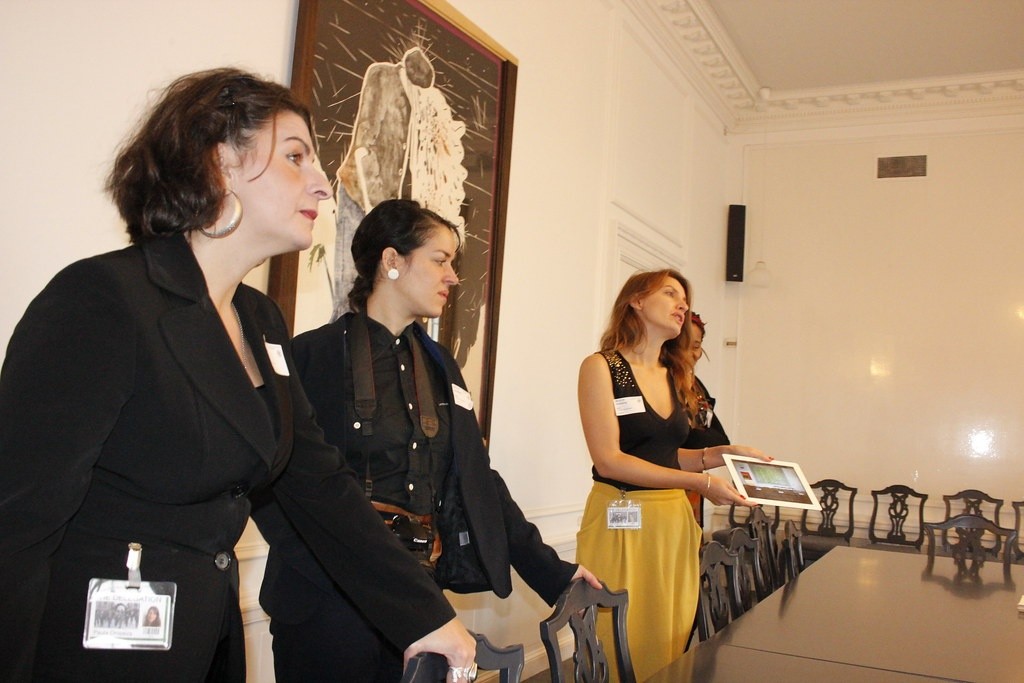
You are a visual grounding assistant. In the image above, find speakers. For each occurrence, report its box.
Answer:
[726,204,746,282]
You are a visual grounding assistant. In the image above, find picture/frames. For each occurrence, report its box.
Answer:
[267,0,520,457]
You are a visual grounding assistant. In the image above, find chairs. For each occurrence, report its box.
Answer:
[728,504,780,540]
[401,577,637,683]
[864,484,1024,563]
[798,479,858,571]
[692,506,805,643]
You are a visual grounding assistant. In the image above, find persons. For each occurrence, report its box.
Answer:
[257,199,604,683]
[678,311,731,527]
[0,65,479,683]
[577,268,777,683]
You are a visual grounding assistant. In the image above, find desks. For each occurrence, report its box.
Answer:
[642,546,1024,683]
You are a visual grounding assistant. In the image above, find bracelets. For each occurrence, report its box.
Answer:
[706,472,711,500]
[702,447,708,471]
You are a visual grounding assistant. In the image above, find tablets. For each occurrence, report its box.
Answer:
[722,452,823,512]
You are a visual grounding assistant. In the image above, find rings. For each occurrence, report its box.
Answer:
[448,662,477,683]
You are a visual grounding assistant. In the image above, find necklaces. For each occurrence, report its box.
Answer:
[232,302,248,371]
